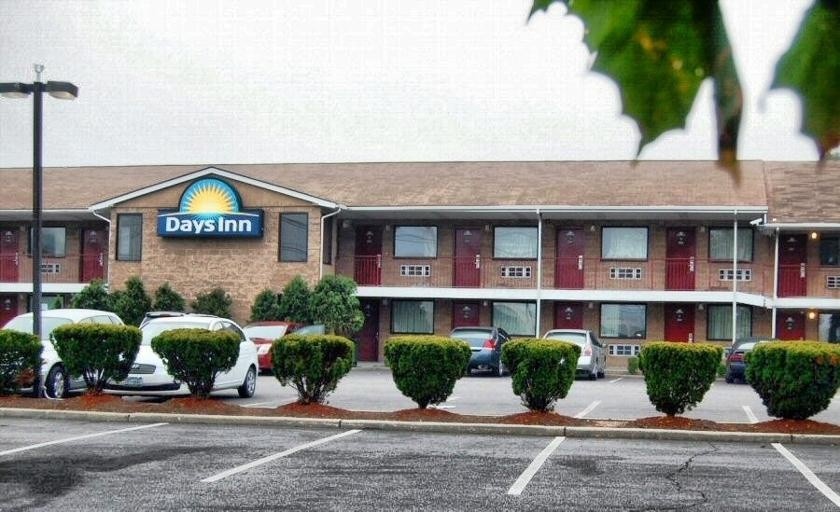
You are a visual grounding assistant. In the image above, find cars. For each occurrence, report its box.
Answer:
[541,329,607,381]
[0,307,128,399]
[241,320,326,375]
[95,316,259,403]
[448,327,513,378]
[723,338,776,384]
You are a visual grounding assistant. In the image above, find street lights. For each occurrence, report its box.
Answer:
[0,62,79,398]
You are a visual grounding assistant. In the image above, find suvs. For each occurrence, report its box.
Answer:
[138,311,222,329]
